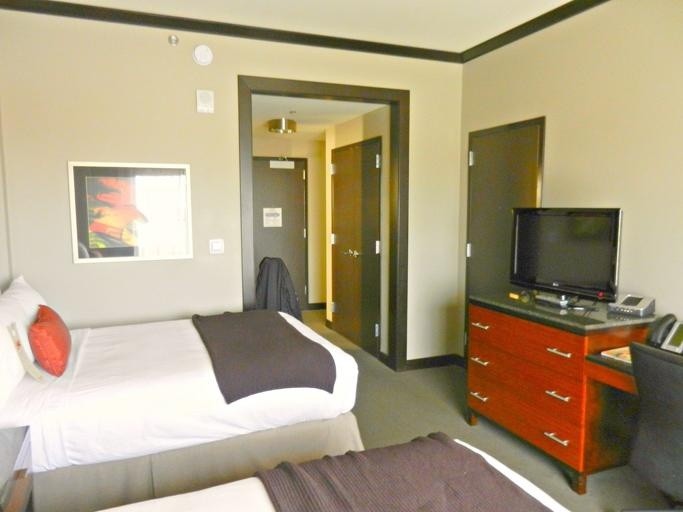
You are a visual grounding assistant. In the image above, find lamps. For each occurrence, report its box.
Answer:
[267,118,297,136]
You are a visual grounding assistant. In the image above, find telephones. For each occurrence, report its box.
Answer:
[648,314,683,355]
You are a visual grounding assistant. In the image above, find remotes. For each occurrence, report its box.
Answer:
[534,296,568,307]
[535,303,568,315]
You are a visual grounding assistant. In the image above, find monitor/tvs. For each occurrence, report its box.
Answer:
[509,208,622,310]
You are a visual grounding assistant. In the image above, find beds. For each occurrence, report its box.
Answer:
[1,274,361,476]
[91,429,574,512]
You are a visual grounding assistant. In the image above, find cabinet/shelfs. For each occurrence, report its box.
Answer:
[466,291,668,493]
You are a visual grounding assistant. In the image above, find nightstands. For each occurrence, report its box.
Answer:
[0,423,35,512]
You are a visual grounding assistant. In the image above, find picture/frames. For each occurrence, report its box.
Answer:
[67,160,195,266]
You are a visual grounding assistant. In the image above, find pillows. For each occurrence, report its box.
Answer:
[26,304,73,377]
[0,275,49,405]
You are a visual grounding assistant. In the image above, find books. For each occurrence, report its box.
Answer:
[600,345,632,365]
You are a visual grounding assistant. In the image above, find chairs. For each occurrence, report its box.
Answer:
[627,342,683,511]
